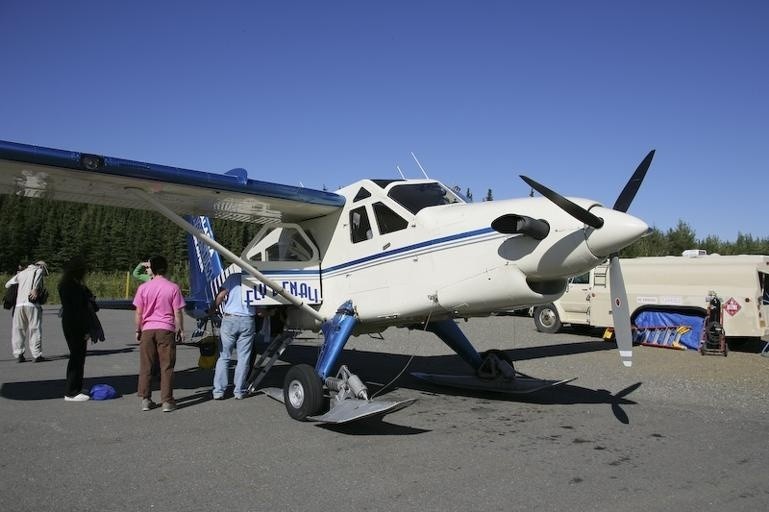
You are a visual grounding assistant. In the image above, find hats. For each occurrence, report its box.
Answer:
[35,261,49,276]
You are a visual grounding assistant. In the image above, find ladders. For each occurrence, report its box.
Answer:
[602,324,693,350]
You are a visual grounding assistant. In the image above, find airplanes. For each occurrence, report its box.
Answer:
[0,139,656,425]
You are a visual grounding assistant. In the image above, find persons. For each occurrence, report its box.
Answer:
[57,255,105,402]
[5,261,49,363]
[207,265,276,401]
[133,255,186,412]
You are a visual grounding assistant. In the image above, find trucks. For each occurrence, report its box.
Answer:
[529,249,769,352]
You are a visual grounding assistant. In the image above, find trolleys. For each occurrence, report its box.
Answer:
[700,303,728,357]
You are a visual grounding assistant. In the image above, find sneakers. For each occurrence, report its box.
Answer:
[16,354,25,362]
[214,395,224,400]
[142,399,157,411]
[162,403,176,412]
[64,393,90,401]
[32,356,45,362]
[234,396,249,400]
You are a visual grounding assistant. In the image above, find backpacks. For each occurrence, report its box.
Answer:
[28,287,48,305]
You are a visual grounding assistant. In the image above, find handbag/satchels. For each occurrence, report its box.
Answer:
[90,384,116,400]
